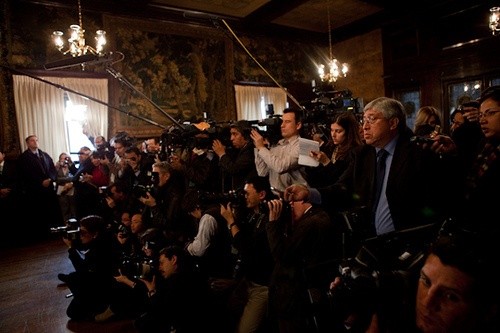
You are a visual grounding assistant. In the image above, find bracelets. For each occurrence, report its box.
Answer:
[46,179,51,183]
[147,289,154,296]
[132,282,136,289]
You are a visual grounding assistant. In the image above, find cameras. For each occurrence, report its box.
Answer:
[117,222,132,238]
[258,196,292,224]
[49,218,81,239]
[98,186,113,200]
[195,187,247,210]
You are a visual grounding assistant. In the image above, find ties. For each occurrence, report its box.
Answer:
[35,152,40,160]
[370,149,390,238]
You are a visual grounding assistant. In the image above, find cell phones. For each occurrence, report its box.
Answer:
[270,187,292,195]
[410,136,447,146]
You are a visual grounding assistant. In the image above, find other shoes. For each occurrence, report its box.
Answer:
[58,273,68,284]
[95,306,116,321]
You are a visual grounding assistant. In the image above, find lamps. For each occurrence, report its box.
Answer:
[51,0,107,71]
[488,7,500,35]
[317,7,351,88]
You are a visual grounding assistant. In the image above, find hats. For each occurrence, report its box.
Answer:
[284,183,323,205]
[138,226,165,244]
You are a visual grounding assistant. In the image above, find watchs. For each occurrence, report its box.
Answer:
[228,222,236,230]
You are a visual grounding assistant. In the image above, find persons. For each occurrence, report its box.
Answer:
[57,98,500,333]
[0,148,16,246]
[18,136,68,240]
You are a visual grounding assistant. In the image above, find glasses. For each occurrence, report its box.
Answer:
[360,116,385,124]
[480,110,500,118]
